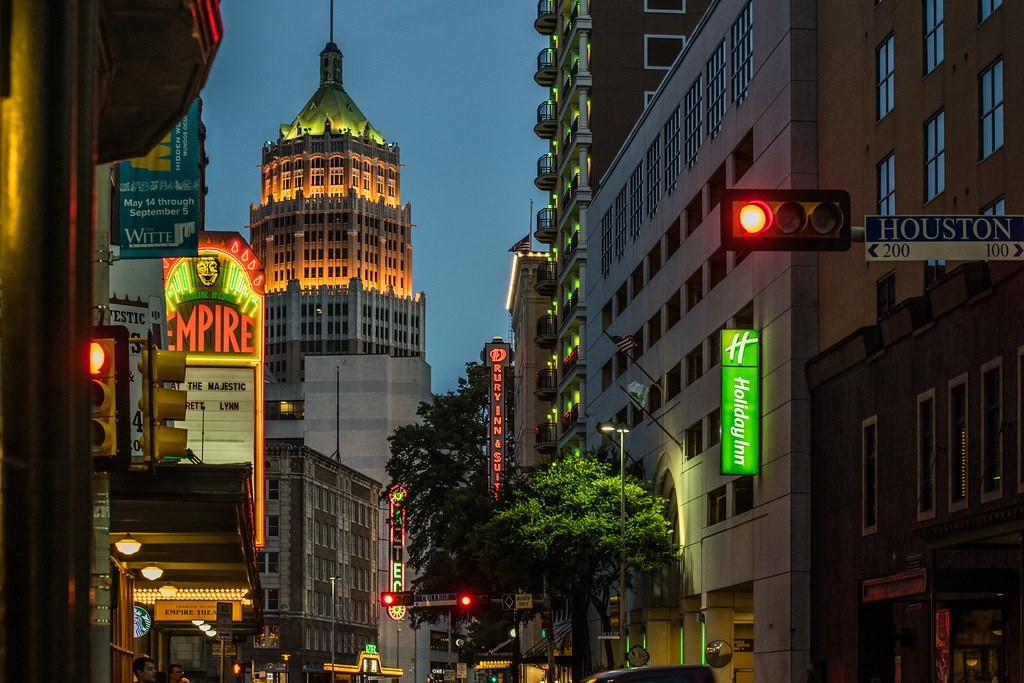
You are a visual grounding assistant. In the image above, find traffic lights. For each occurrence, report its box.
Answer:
[90,324,131,472]
[138,344,188,461]
[457,591,491,611]
[491,671,498,683]
[381,592,414,606]
[720,189,851,251]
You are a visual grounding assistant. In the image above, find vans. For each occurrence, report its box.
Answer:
[580,665,720,683]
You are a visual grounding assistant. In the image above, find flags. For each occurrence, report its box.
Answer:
[624,381,650,410]
[610,334,635,352]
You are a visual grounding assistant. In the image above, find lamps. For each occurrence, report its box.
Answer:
[198,623,211,631]
[192,620,204,626]
[205,628,217,637]
[158,582,178,597]
[114,532,142,555]
[141,563,163,581]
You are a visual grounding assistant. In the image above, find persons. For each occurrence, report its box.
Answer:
[133,657,157,683]
[168,664,190,683]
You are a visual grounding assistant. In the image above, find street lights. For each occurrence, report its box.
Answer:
[600,422,635,683]
[330,576,337,683]
